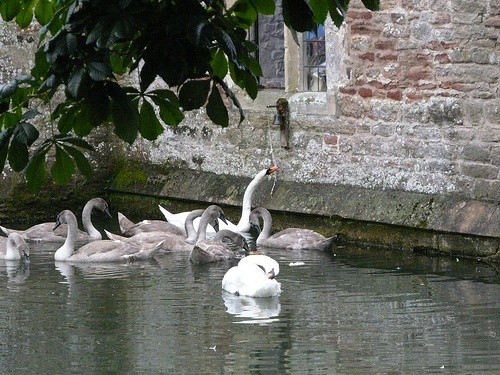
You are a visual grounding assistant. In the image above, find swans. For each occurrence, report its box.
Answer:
[103,203,229,254]
[221,253,283,298]
[51,209,167,262]
[0,232,31,264]
[187,228,249,265]
[116,209,222,245]
[248,207,340,250]
[157,164,280,236]
[0,197,113,241]
